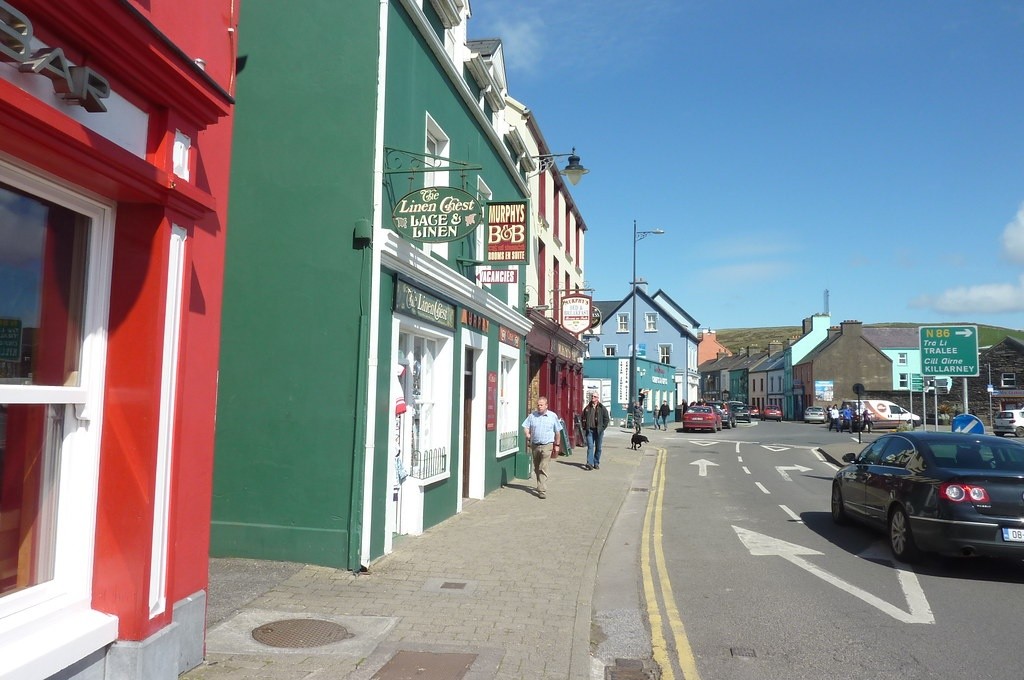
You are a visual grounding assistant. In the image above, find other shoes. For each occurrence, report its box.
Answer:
[586,463,593,469]
[539,492,546,499]
[594,465,599,469]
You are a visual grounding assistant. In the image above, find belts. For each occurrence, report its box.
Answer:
[534,443,546,446]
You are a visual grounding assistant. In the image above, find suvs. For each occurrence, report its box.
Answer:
[761,404,783,421]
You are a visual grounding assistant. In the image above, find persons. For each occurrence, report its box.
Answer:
[826,404,840,433]
[841,404,853,434]
[972,410,976,415]
[860,410,872,434]
[521,397,564,499]
[653,405,661,429]
[658,400,670,431]
[682,397,707,415]
[633,401,643,434]
[581,392,610,471]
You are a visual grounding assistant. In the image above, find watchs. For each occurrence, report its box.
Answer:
[555,444,560,446]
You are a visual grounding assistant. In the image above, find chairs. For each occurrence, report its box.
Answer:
[936,456,956,467]
[958,449,984,468]
[995,460,1024,471]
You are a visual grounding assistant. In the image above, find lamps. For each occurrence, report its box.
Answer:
[525,145,590,187]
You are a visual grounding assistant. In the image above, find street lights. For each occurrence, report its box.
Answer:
[627,219,664,432]
[984,362,993,427]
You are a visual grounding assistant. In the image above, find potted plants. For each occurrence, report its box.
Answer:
[937,405,949,414]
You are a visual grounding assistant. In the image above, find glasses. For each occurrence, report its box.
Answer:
[591,395,596,398]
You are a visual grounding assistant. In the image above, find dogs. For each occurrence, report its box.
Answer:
[630,432,649,450]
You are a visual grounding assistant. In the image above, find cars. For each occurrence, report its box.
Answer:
[831,430,1024,563]
[993,410,1024,437]
[683,400,759,433]
[804,406,828,424]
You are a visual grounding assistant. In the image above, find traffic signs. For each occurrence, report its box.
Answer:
[919,325,979,377]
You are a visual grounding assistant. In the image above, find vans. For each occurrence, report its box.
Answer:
[839,399,922,430]
